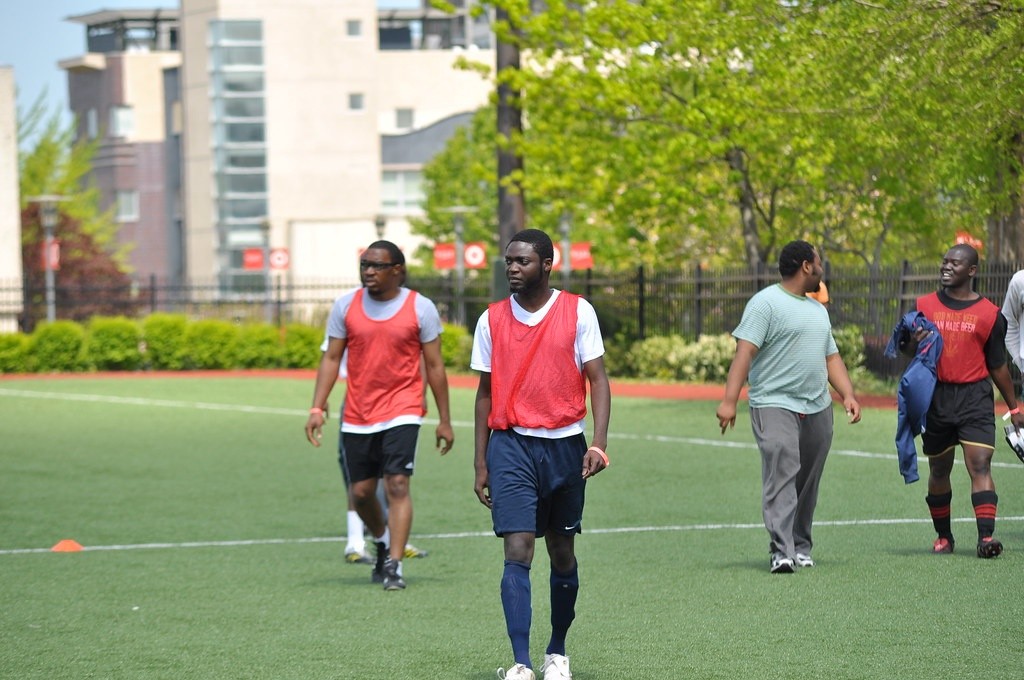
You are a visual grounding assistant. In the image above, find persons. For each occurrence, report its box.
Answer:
[1001,269,1024,403]
[305,240,455,590]
[320,250,444,563]
[898,244,1024,558]
[470,229,611,680]
[804,280,829,309]
[716,240,862,574]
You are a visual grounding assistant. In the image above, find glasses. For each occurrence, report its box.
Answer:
[362,261,394,272]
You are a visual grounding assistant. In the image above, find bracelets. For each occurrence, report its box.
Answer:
[311,408,323,414]
[588,446,609,466]
[1002,407,1020,421]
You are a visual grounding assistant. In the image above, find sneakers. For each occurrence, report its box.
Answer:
[369,540,390,584]
[401,543,429,559]
[769,552,793,573]
[497,664,536,680]
[383,556,406,589]
[1004,425,1024,462]
[974,537,1005,557]
[797,552,815,566]
[540,653,572,680]
[930,534,956,556]
[344,549,375,564]
